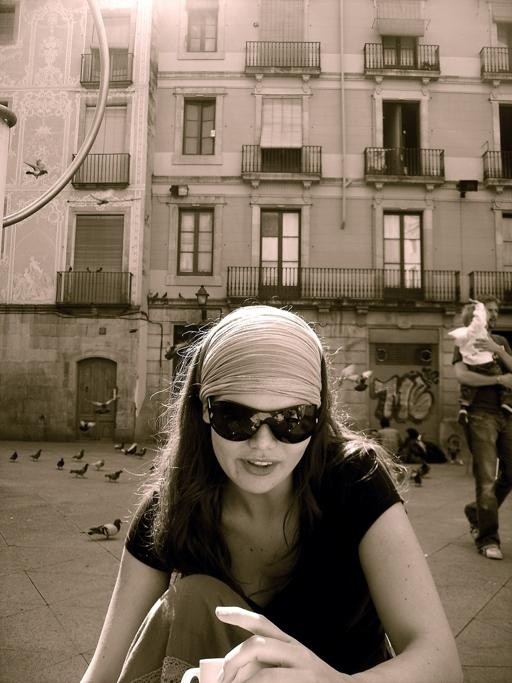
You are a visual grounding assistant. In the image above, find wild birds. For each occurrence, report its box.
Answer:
[86,266,89,270]
[69,266,72,270]
[96,267,102,272]
[161,292,167,299]
[179,293,183,298]
[152,292,158,298]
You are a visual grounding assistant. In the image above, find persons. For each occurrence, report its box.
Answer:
[370,429,379,436]
[449,297,511,423]
[402,428,446,462]
[76,303,463,683]
[452,296,511,562]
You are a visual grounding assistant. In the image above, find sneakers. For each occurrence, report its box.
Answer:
[458,409,469,425]
[480,543,503,560]
[501,404,512,415]
[470,522,480,539]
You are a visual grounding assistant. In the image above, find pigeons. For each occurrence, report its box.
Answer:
[87,518,123,539]
[23,159,48,179]
[410,460,430,487]
[79,420,97,432]
[347,370,375,391]
[164,341,190,360]
[90,193,113,206]
[84,397,121,414]
[111,440,147,456]
[6,449,122,483]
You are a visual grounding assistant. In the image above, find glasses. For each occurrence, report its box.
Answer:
[207,397,322,444]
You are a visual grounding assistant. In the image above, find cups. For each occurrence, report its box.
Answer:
[180,658,228,682]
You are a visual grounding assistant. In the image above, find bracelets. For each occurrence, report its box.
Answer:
[496,375,502,385]
